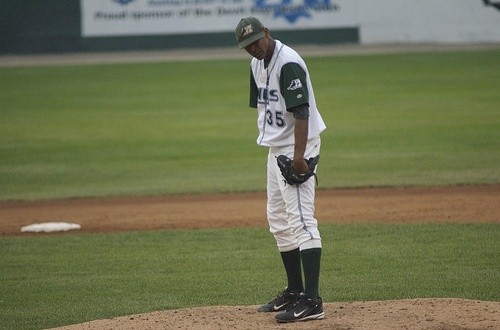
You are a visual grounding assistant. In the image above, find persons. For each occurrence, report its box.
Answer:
[236,17,326,323]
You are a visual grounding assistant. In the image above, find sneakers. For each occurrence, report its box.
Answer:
[275,292,325,322]
[256,286,305,313]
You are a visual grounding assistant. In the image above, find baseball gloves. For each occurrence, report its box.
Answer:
[276,153,320,187]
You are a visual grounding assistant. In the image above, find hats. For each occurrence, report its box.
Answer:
[235,16,264,49]
